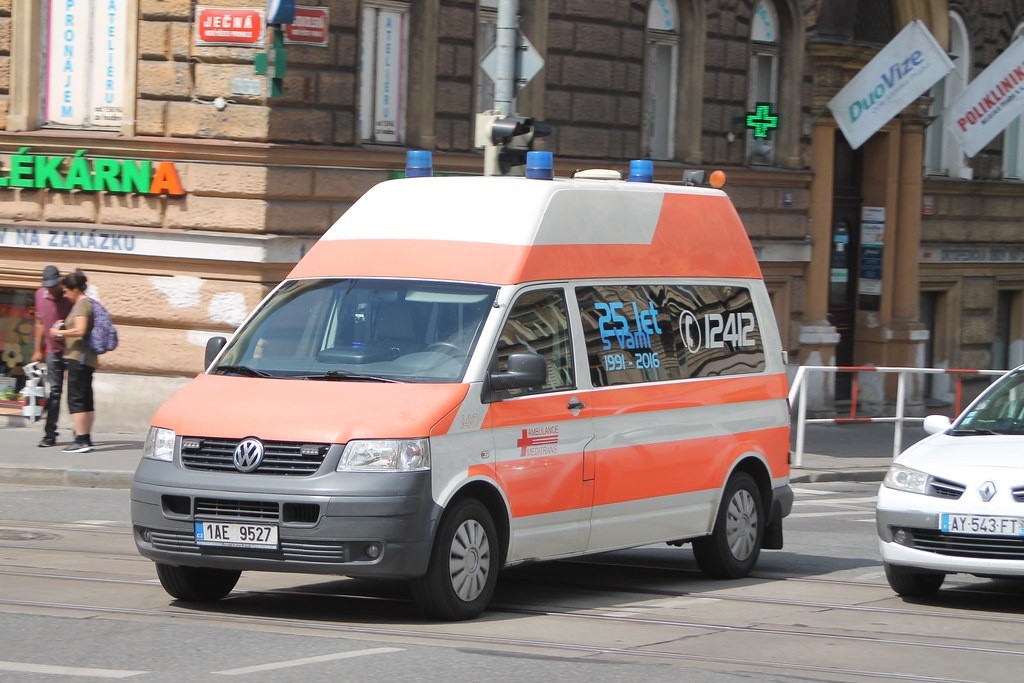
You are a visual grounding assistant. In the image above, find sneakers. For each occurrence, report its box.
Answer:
[61,443,92,453]
[39,433,58,446]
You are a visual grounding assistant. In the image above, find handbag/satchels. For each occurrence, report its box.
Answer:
[84,298,118,354]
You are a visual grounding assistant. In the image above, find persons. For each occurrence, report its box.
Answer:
[49,270,99,453]
[31,266,76,448]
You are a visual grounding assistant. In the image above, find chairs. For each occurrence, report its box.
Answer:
[372,300,429,355]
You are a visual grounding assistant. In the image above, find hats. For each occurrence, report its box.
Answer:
[42,265,59,287]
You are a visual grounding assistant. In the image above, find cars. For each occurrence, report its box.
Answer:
[872,363,1023,603]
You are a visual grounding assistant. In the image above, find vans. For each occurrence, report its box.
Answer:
[128,142,795,625]
